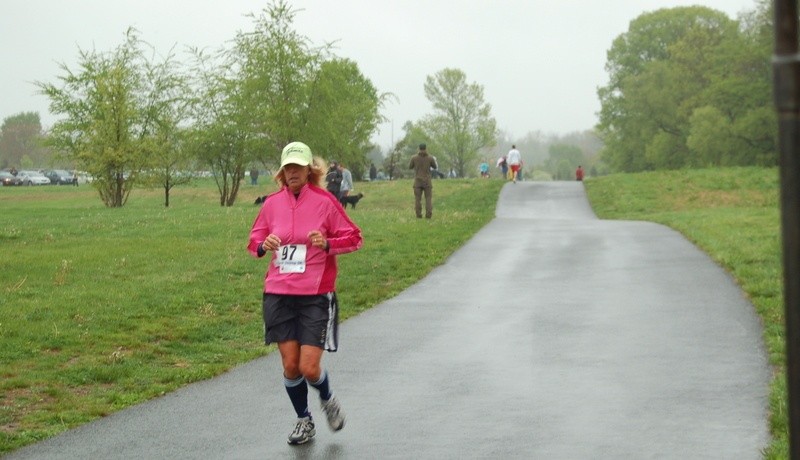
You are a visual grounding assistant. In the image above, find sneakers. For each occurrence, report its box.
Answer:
[286,413,316,444]
[319,392,346,432]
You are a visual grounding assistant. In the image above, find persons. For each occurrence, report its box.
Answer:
[10,167,18,177]
[238,144,584,219]
[247,141,360,446]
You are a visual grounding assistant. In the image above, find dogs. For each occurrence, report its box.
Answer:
[341,193,364,209]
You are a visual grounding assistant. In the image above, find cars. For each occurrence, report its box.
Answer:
[0,168,93,186]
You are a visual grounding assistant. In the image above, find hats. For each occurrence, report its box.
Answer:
[280,141,313,167]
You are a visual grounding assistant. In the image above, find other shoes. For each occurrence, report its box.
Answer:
[513,179,516,184]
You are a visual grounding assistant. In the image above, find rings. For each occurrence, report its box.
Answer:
[315,239,316,242]
